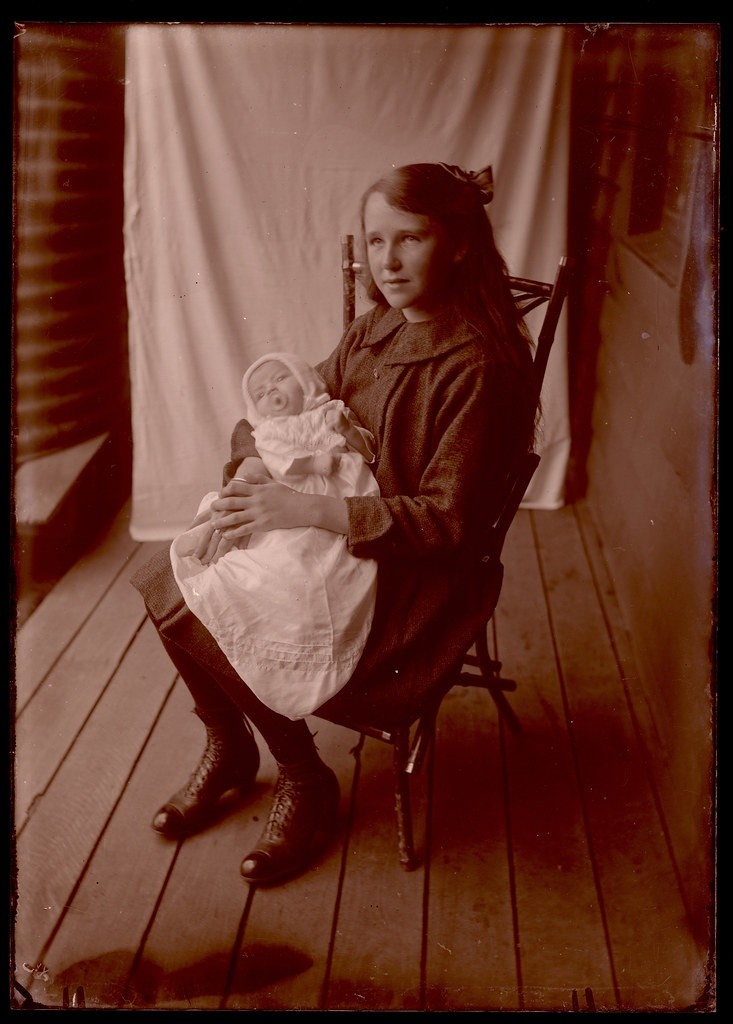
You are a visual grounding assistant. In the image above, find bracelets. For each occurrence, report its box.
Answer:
[230,477,249,484]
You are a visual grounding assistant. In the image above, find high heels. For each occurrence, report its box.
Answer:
[149,720,259,839]
[239,750,341,883]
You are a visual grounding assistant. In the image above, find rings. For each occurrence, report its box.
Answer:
[211,526,223,536]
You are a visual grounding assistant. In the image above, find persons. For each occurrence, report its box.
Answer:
[124,162,546,889]
[241,350,385,602]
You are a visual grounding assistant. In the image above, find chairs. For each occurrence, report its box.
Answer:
[316,236,572,870]
